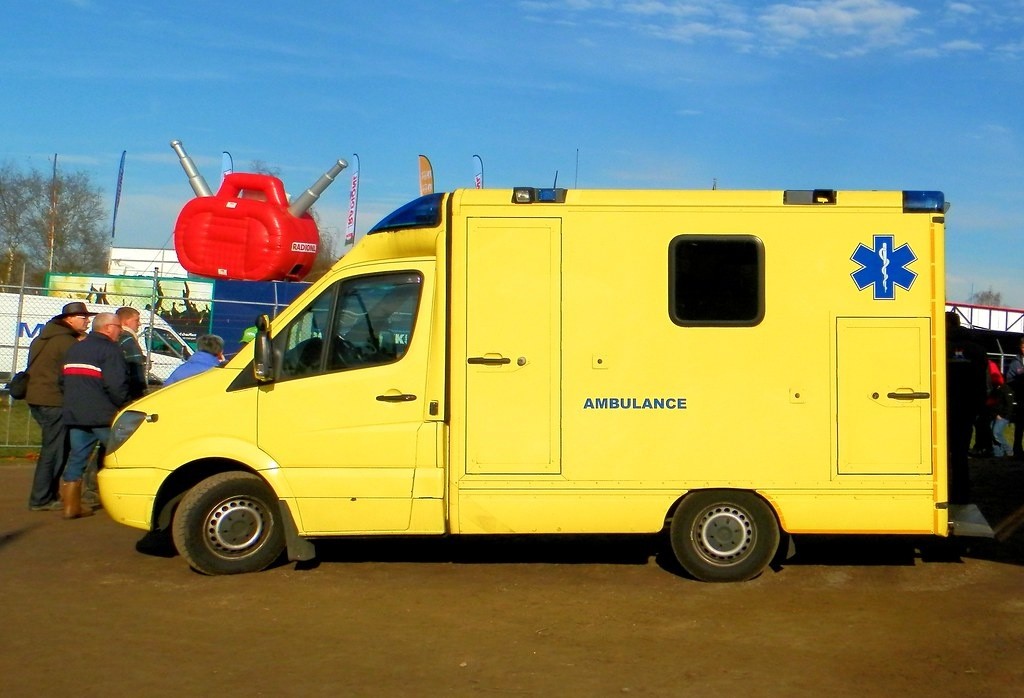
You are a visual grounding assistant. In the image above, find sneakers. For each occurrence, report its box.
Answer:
[32,500,63,511]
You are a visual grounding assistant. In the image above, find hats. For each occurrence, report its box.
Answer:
[52,302,99,319]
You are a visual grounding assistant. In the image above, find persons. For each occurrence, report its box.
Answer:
[25,302,147,519]
[161,334,226,387]
[943,312,989,460]
[1006,338,1024,382]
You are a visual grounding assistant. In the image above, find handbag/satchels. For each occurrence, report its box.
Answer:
[10,372,30,399]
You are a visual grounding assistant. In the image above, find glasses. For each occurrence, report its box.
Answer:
[74,315,88,319]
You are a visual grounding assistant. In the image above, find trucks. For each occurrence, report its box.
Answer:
[0,292,196,381]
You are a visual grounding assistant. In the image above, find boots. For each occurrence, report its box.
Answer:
[61,480,96,518]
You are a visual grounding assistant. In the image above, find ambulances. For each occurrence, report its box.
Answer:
[96,189,955,583]
